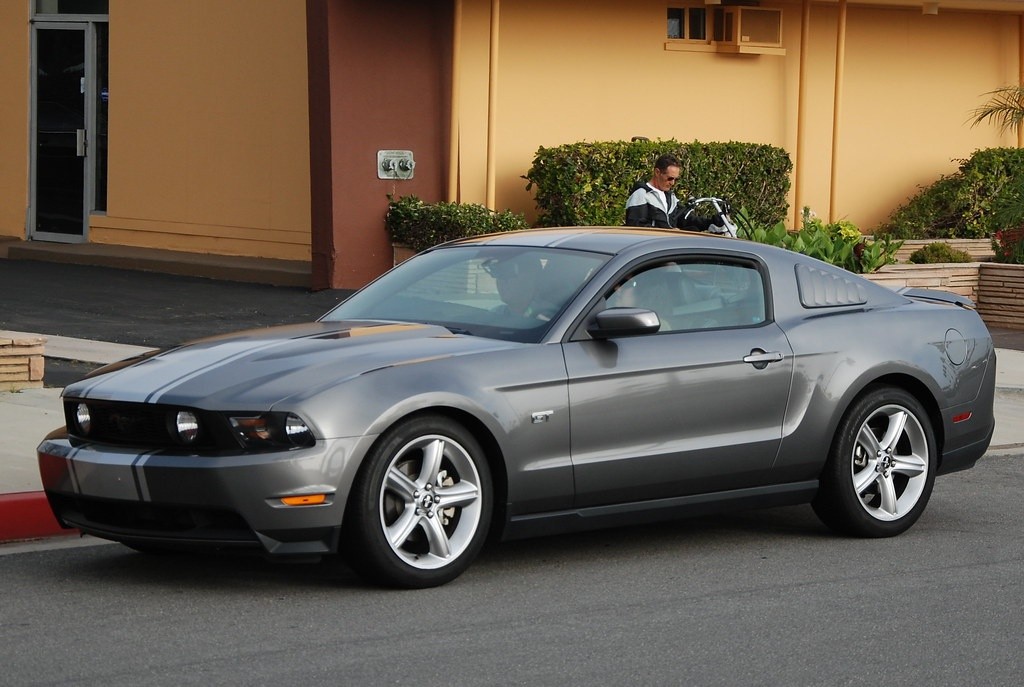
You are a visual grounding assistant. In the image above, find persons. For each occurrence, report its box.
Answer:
[626,154,712,232]
[491,257,671,332]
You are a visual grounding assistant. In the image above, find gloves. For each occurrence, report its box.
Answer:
[711,211,726,227]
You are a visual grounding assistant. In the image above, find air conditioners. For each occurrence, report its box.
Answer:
[716,5,784,47]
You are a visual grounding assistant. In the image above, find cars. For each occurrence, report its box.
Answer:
[36,224,998,591]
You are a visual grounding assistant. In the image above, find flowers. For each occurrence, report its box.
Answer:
[747,207,905,276]
[989,227,1024,264]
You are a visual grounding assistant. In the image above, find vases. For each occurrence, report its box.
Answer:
[861,272,910,292]
[976,264,1024,331]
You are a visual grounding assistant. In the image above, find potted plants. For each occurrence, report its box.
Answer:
[385,191,533,296]
[880,240,980,310]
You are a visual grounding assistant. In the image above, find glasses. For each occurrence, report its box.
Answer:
[619,279,640,289]
[661,173,679,182]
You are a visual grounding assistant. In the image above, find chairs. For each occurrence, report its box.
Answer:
[641,271,719,331]
